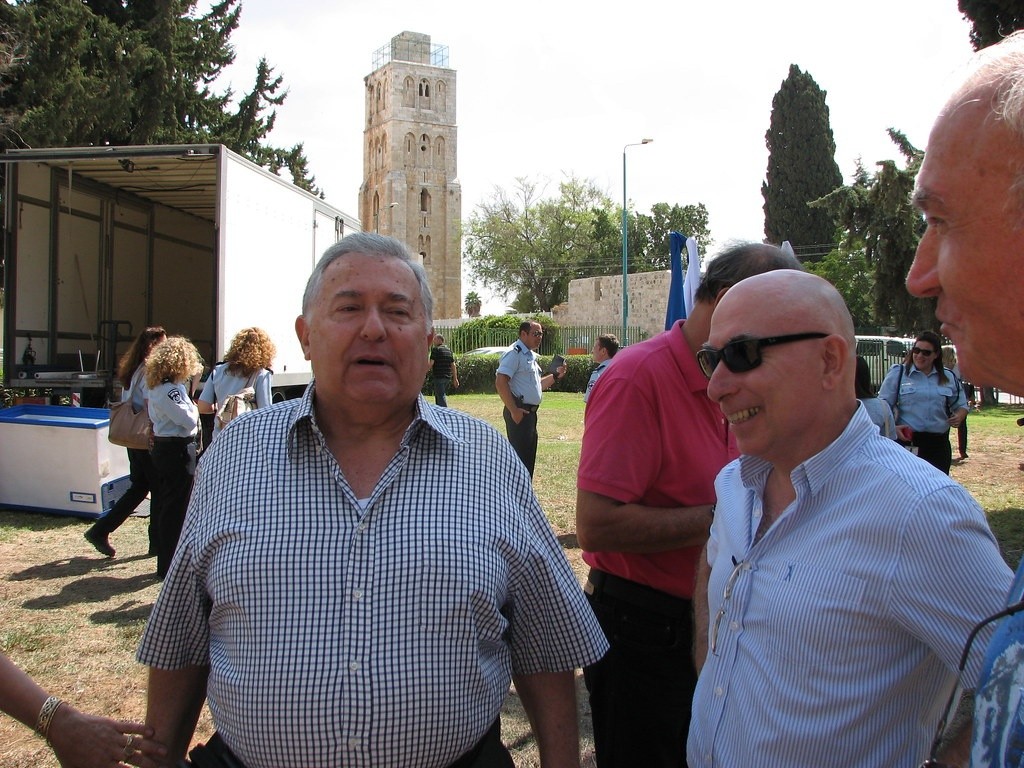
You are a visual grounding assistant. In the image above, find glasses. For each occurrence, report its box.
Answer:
[694,331,842,377]
[910,346,934,356]
[527,330,544,336]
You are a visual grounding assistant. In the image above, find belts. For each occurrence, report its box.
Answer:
[584,565,693,622]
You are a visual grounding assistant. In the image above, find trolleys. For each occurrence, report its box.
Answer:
[95,320,133,411]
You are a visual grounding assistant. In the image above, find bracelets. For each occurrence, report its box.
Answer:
[553,374,559,382]
[35,695,70,739]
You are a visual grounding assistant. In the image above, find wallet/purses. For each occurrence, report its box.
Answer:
[550,354,565,375]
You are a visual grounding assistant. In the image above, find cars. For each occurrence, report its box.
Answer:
[462,346,540,357]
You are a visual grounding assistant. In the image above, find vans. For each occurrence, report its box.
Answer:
[853,335,917,388]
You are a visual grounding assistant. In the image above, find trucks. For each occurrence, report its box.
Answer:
[1,144,362,411]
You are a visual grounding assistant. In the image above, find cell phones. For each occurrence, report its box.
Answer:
[902,427,912,440]
[548,354,565,375]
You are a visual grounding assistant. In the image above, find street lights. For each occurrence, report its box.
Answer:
[621,137,655,348]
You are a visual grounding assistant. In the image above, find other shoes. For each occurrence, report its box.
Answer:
[959,451,970,459]
[84,524,123,557]
[156,567,170,578]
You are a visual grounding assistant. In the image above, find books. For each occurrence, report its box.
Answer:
[550,354,566,376]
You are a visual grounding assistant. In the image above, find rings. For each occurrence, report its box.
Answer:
[126,735,136,746]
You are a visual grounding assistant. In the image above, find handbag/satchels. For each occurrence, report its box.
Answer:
[215,386,261,430]
[890,405,901,424]
[108,397,154,451]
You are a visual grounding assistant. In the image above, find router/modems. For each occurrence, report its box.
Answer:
[72,350,102,379]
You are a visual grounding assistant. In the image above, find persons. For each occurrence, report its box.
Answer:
[573,244,809,768]
[855,331,976,476]
[495,320,567,479]
[692,270,1015,768]
[134,233,610,768]
[427,334,459,407]
[583,334,619,404]
[906,30,1024,768]
[84,326,275,579]
[0,651,167,768]
